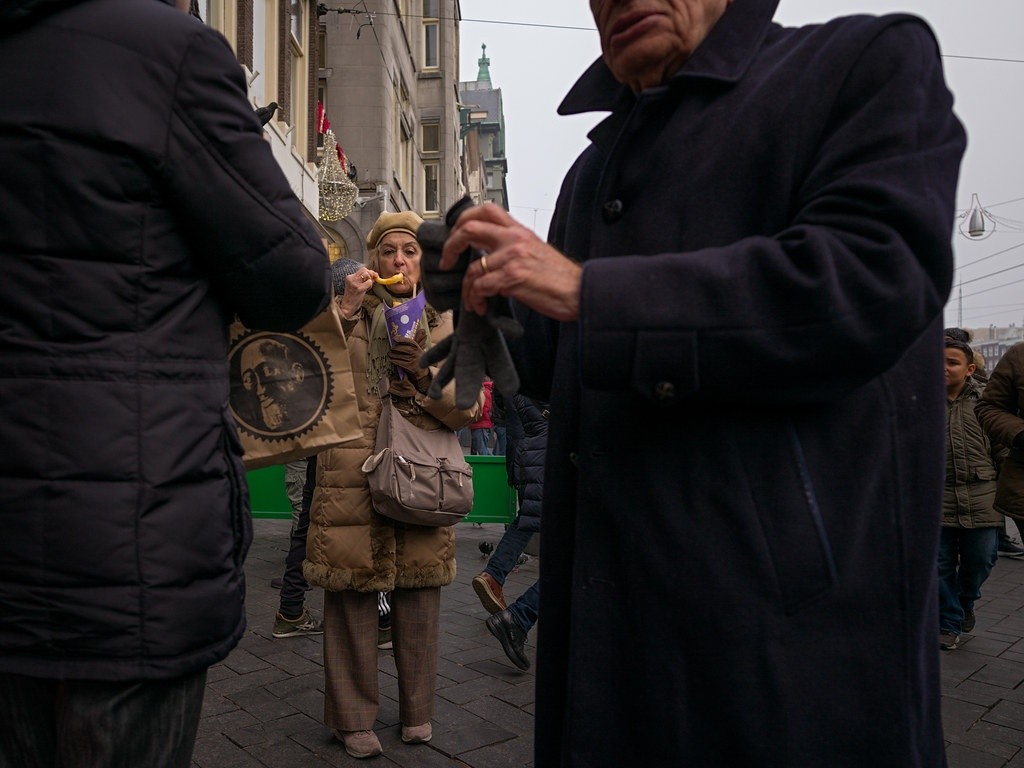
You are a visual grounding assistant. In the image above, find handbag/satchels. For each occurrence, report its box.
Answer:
[229,298,363,470]
[362,406,474,526]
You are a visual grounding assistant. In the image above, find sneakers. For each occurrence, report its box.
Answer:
[272,609,324,637]
[472,571,507,615]
[486,609,536,670]
[939,630,960,649]
[335,729,383,757]
[402,722,432,743]
[997,536,1024,556]
[958,595,976,633]
[377,627,393,649]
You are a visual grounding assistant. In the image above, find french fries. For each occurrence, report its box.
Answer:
[375,273,403,285]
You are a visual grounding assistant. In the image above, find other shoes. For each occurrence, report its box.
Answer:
[271,577,314,591]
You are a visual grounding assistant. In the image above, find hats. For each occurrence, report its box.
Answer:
[365,210,425,252]
[331,258,364,295]
[972,351,985,368]
[943,328,974,344]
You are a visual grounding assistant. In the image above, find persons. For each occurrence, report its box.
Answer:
[0,0,334,768]
[939,328,1005,649]
[274,455,393,649]
[974,342,1024,544]
[440,0,968,768]
[469,382,545,673]
[305,211,486,758]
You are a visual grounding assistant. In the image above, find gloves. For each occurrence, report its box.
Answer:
[416,195,523,409]
[386,329,433,397]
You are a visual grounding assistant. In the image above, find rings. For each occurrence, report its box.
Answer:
[481,256,490,274]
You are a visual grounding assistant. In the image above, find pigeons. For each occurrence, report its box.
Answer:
[255,102,284,126]
[479,541,494,555]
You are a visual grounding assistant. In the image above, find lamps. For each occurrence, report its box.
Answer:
[968,202,986,239]
[459,111,489,139]
